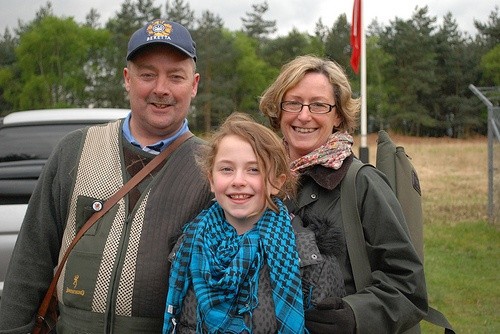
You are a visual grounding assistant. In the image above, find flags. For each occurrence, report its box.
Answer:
[350,0,361,74]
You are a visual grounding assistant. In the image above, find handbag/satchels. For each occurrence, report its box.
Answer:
[32,295,58,334]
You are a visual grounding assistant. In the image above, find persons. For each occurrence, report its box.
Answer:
[258,55,428,334]
[164,113,348,334]
[0,19,214,334]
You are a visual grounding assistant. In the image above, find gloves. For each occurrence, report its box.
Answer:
[306,296,357,334]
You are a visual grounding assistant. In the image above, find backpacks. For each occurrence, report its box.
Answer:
[341,129,424,291]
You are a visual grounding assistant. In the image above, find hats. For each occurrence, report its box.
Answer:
[126,19,197,64]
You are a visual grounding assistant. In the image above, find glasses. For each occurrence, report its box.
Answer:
[280,100,337,114]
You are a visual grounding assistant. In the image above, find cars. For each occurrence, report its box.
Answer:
[0,108,132,301]
